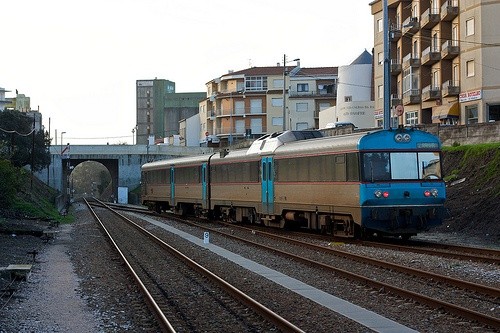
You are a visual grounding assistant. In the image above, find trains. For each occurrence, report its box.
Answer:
[140,124,446,241]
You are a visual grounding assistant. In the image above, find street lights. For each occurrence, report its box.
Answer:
[282,53,300,131]
